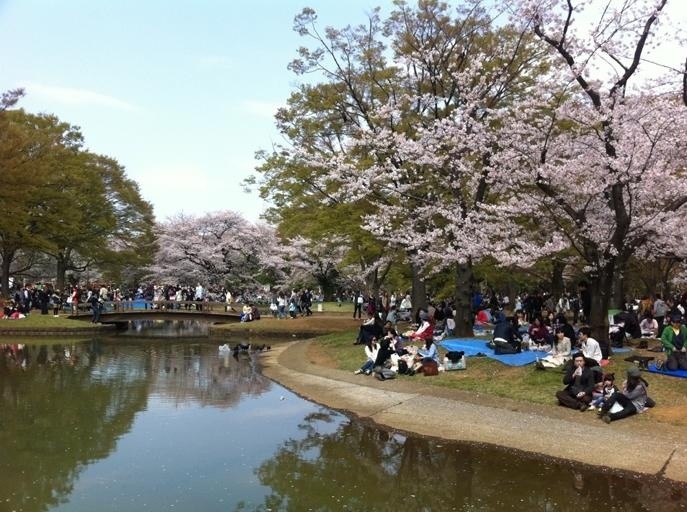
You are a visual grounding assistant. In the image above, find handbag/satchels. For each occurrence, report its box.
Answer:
[569,385,592,403]
[422,361,438,376]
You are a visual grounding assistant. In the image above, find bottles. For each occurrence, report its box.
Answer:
[575,472,581,483]
[641,360,645,368]
[577,364,582,376]
[535,355,538,363]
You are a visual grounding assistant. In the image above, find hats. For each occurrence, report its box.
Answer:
[627,365,642,377]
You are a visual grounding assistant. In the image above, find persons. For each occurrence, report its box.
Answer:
[556,327,648,423]
[144,342,201,380]
[337,288,457,381]
[568,467,589,497]
[471,290,579,368]
[609,293,686,371]
[1,339,106,373]
[0,281,314,322]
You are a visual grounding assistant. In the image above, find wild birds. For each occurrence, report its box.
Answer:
[218,343,271,352]
